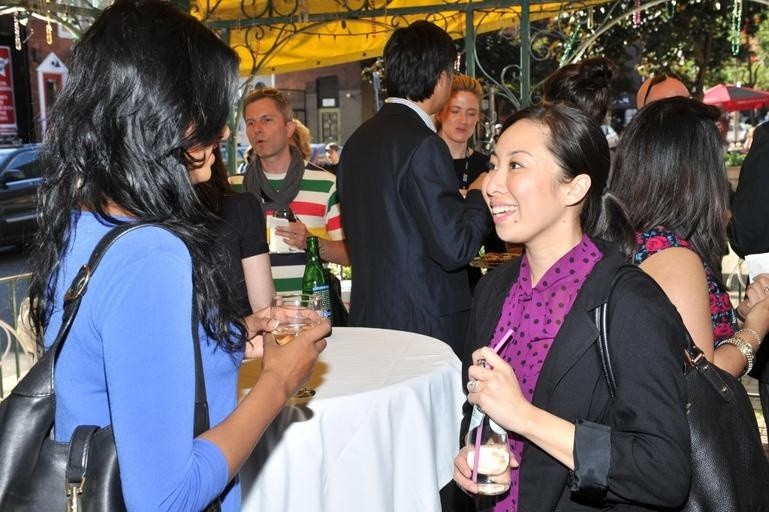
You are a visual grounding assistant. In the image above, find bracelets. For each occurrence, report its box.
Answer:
[717,327,761,380]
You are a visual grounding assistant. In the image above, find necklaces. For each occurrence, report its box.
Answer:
[460,146,471,190]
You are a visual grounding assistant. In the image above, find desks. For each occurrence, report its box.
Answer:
[232,324,465,512]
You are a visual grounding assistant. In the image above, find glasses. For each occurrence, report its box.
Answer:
[642,71,684,106]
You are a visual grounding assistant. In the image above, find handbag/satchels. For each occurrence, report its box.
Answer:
[1,221,224,511]
[588,263,769,512]
[323,269,350,327]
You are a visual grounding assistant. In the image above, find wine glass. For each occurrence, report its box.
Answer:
[270,296,322,399]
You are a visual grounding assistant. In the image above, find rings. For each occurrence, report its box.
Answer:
[764,288,769,293]
[466,379,480,393]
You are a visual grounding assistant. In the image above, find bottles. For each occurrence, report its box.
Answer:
[300,236,332,337]
[466,360,512,495]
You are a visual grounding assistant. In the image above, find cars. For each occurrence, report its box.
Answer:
[0,142,46,248]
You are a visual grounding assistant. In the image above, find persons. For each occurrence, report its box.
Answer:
[430,71,508,300]
[192,143,279,359]
[228,87,353,308]
[27,1,334,511]
[290,116,329,174]
[609,96,769,380]
[530,57,620,192]
[335,20,496,361]
[726,118,769,449]
[315,142,339,177]
[439,104,694,512]
